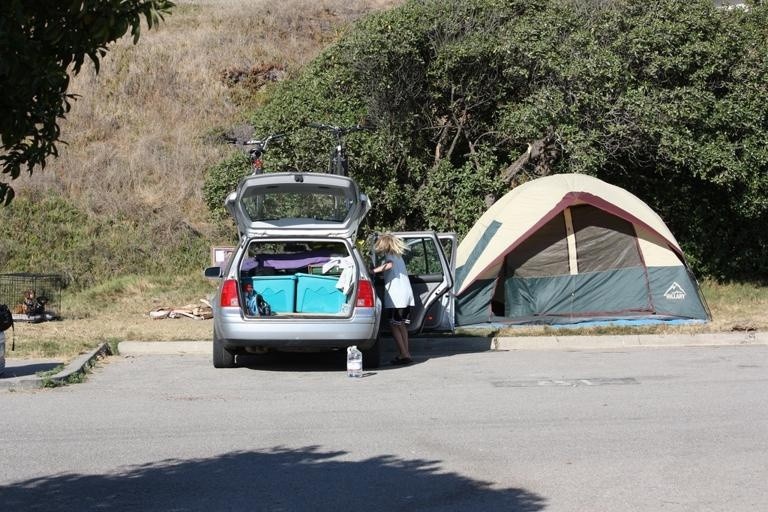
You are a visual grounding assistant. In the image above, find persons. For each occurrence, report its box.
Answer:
[369,234,416,365]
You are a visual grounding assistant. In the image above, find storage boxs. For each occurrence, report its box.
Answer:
[241,274,297,313]
[295,273,347,314]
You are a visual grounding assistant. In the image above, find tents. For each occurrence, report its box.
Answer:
[439,172,713,328]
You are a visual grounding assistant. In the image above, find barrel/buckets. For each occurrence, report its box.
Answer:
[346,345,363,378]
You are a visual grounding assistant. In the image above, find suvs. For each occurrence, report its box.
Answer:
[201,165,462,364]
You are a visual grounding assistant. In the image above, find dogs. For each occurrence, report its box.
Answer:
[13,288,31,313]
[27,296,48,315]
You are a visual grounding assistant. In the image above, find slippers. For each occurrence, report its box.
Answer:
[391,355,411,364]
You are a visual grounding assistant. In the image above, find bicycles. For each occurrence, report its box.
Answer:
[225,128,294,216]
[297,116,383,223]
